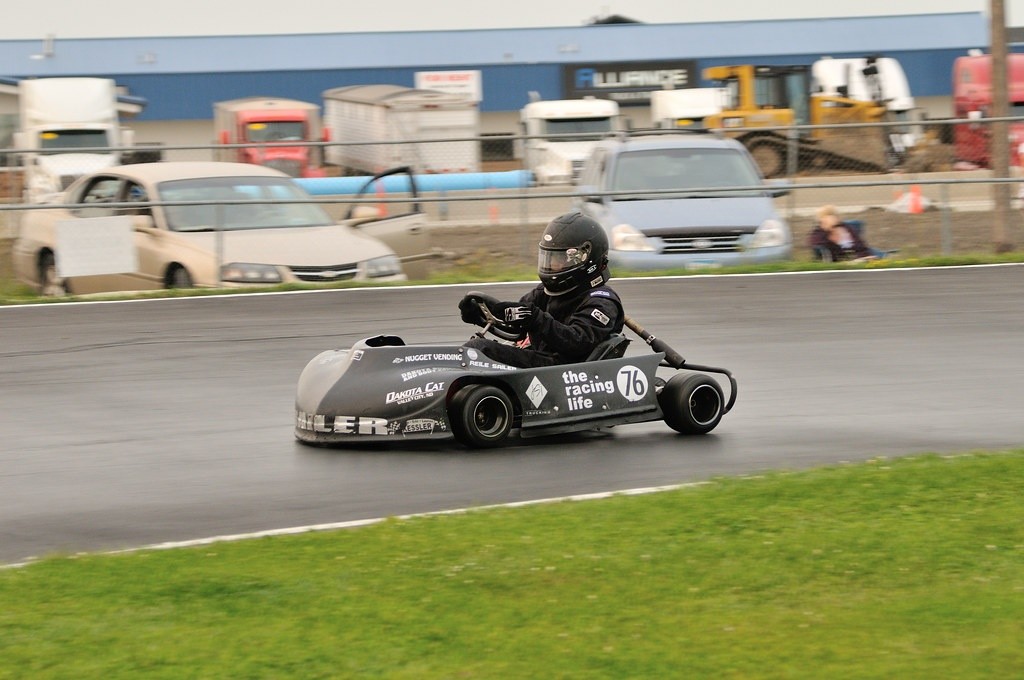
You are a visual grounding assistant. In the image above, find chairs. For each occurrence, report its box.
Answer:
[585,333,634,361]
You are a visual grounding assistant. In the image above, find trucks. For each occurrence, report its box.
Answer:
[211,96,331,179]
[12,77,120,183]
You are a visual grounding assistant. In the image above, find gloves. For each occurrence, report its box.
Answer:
[458,295,483,324]
[491,302,540,332]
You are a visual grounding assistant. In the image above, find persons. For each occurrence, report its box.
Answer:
[458,210,627,363]
[808,207,873,262]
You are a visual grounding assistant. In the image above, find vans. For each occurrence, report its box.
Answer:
[518,99,624,183]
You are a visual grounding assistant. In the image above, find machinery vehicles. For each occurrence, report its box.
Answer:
[675,65,941,177]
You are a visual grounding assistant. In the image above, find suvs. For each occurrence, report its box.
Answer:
[571,129,794,271]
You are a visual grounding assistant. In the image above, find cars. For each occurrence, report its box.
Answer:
[13,161,433,294]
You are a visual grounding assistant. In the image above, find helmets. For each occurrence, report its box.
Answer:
[537,212,610,301]
